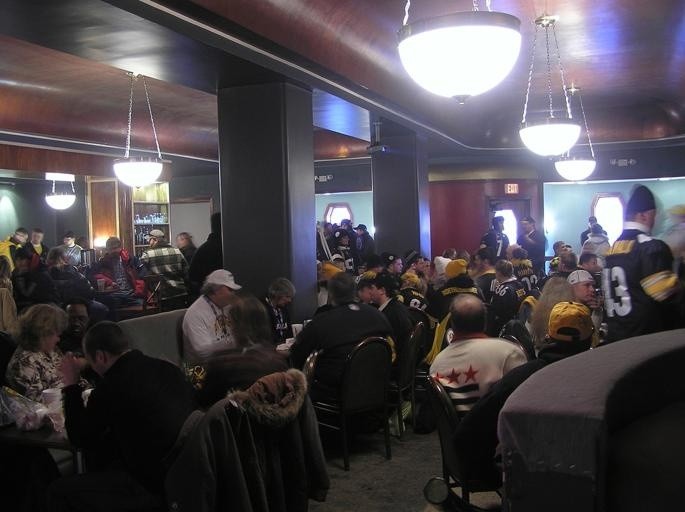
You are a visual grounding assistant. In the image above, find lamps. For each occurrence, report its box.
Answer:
[555,82,599,183]
[513,1,581,162]
[396,0,524,104]
[111,67,169,191]
[43,169,81,212]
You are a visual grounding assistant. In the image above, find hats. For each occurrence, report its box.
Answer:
[566,271,596,286]
[548,300,595,343]
[445,259,468,279]
[144,230,165,240]
[353,224,367,232]
[381,252,399,267]
[330,254,345,262]
[207,268,241,291]
[625,185,656,213]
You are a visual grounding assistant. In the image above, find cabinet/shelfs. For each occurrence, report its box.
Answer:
[87,173,173,260]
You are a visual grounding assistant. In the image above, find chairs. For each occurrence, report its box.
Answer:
[312,333,395,471]
[420,375,504,512]
[390,322,425,442]
[143,395,257,512]
[173,312,193,373]
[240,369,332,512]
[241,243,608,385]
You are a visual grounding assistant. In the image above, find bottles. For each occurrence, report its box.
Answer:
[134,225,149,245]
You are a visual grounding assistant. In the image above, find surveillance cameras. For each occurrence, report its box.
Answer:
[368,144,387,154]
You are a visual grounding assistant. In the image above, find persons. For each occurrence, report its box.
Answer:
[1,185,684,511]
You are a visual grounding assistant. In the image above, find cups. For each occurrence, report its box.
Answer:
[41,388,60,405]
[304,320,312,326]
[97,278,105,293]
[291,324,304,337]
[286,338,293,343]
